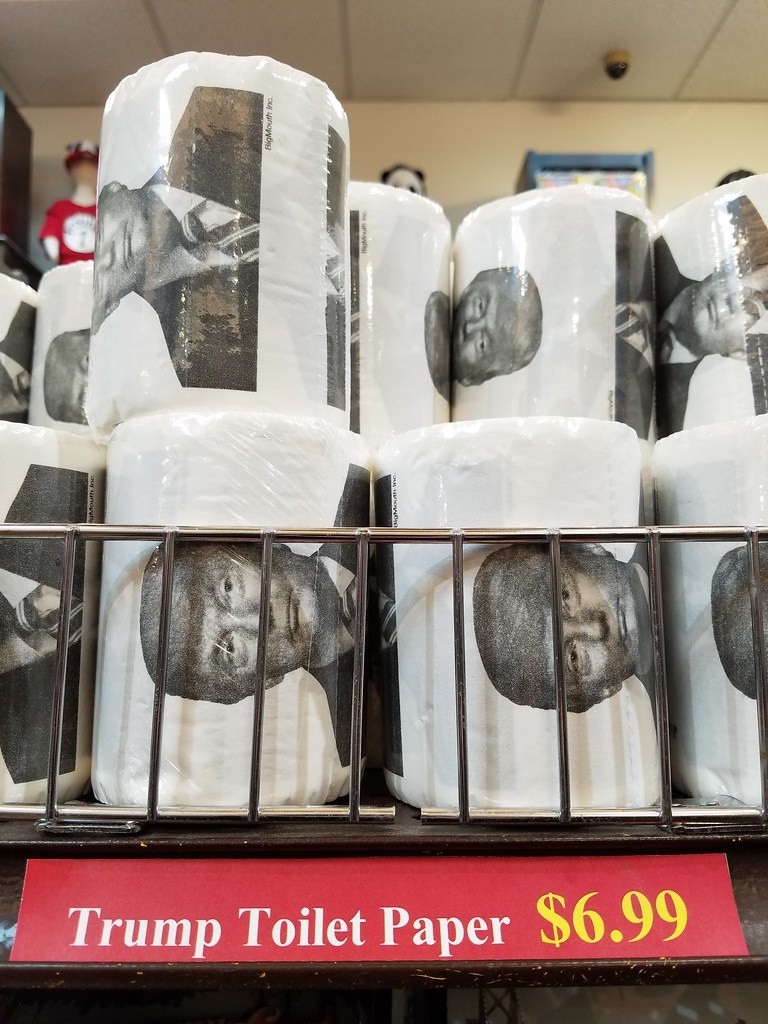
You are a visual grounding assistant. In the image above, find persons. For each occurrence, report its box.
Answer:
[88,84,264,393]
[140,466,370,767]
[42,329,91,426]
[450,266,542,387]
[653,234,768,438]
[472,542,659,737]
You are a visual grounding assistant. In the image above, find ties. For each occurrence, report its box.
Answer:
[11,376,32,405]
[614,302,652,355]
[741,290,767,320]
[178,209,259,263]
[336,589,397,645]
[11,596,83,648]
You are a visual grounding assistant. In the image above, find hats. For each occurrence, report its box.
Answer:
[63,141,99,165]
